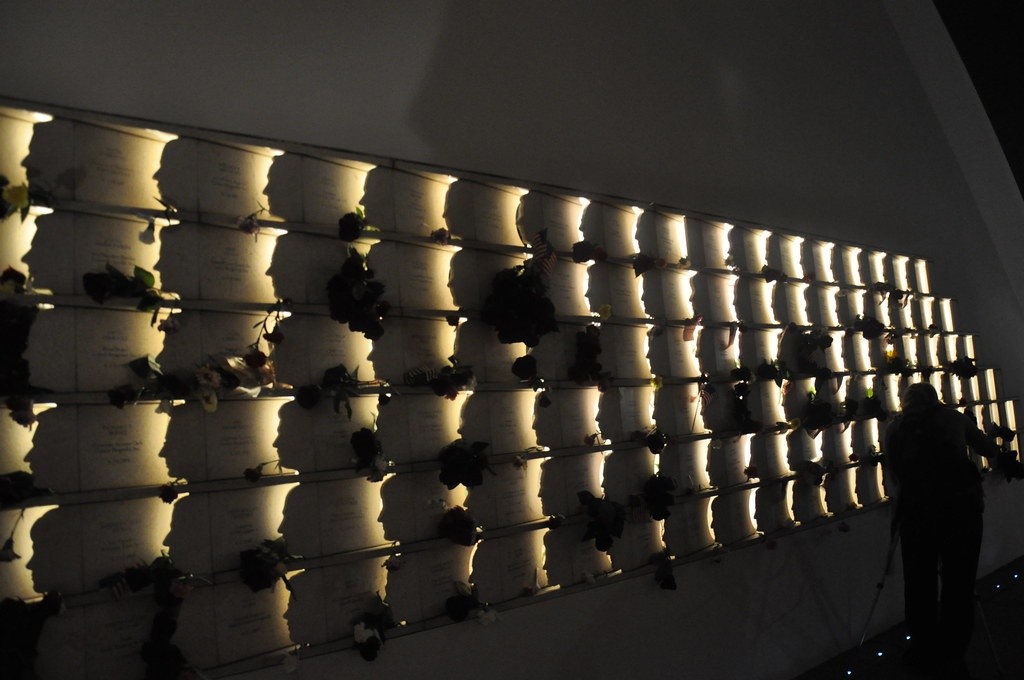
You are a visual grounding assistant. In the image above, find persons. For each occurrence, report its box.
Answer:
[882,383,1024,679]
